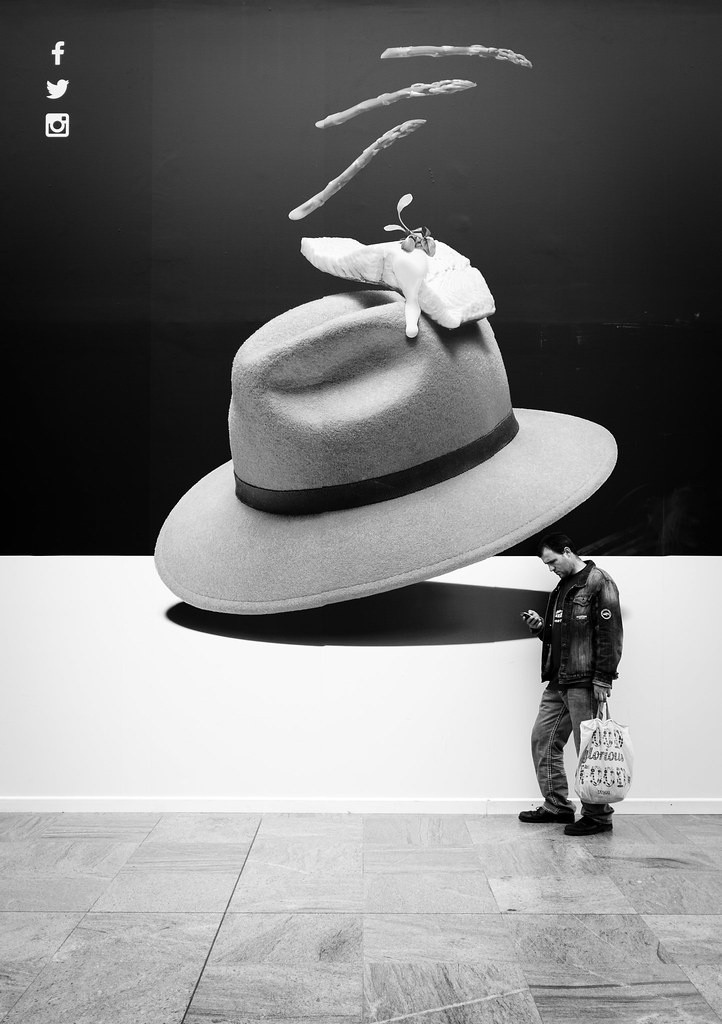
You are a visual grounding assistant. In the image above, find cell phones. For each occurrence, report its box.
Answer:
[520,612,538,625]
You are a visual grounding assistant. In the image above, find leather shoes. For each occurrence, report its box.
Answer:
[519,807,577,824]
[565,816,613,835]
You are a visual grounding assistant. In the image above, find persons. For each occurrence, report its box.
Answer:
[519,530,625,838]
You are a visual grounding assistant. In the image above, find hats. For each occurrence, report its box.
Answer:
[154,293,621,625]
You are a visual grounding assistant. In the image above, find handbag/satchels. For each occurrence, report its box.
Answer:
[575,700,632,805]
